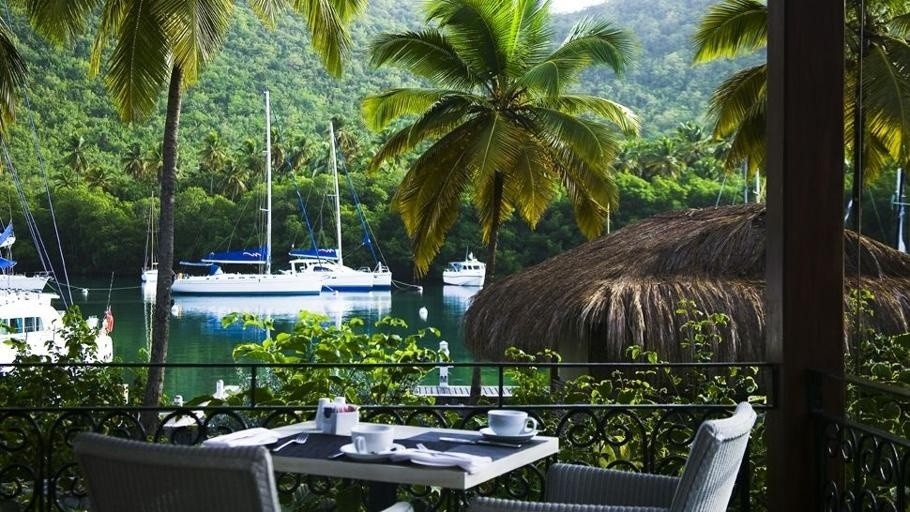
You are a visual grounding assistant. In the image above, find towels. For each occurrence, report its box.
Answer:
[202,426,279,448]
[391,447,492,475]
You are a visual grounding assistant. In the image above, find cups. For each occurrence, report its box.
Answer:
[350,425,394,454]
[488,409,538,436]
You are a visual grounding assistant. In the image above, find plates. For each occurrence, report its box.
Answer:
[339,442,405,460]
[480,427,538,442]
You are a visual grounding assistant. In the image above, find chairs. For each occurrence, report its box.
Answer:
[469,400,757,512]
[75,432,417,512]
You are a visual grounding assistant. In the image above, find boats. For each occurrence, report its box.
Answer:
[443,246,486,286]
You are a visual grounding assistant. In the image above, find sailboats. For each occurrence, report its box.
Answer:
[0,90,113,376]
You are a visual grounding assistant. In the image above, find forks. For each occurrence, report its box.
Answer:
[272,432,309,454]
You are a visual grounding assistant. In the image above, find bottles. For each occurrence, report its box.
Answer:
[315,397,346,430]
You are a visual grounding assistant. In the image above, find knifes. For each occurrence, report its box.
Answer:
[439,437,522,449]
